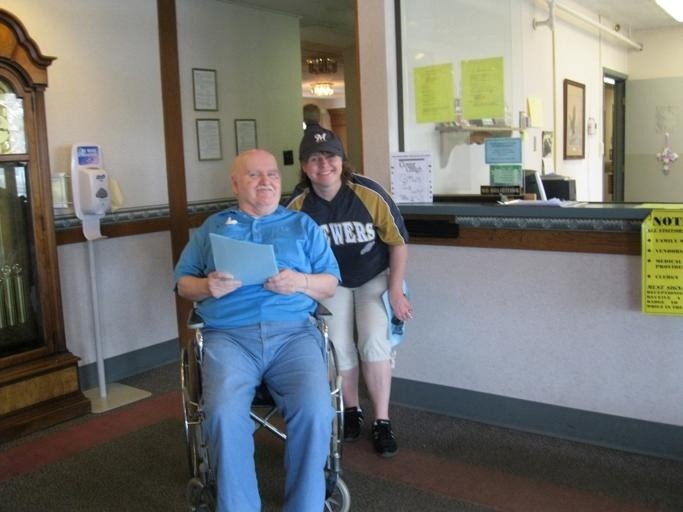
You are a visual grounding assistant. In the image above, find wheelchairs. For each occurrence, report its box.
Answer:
[175,298,353,512]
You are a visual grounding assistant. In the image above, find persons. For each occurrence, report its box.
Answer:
[302,104,327,135]
[173,148,343,512]
[282,125,413,459]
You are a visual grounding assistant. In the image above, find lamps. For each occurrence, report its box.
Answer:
[307,56,337,73]
[312,83,334,96]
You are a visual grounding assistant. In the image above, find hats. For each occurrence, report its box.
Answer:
[299,129,343,162]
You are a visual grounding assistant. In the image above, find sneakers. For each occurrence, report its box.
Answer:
[370,419,398,458]
[343,406,367,442]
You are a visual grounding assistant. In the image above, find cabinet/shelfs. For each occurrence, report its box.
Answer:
[0,7,92,443]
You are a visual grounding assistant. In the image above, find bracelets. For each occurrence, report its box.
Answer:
[302,273,310,294]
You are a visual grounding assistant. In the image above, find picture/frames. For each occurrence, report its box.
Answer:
[191,67,219,112]
[235,118,258,156]
[563,79,585,160]
[196,118,223,161]
